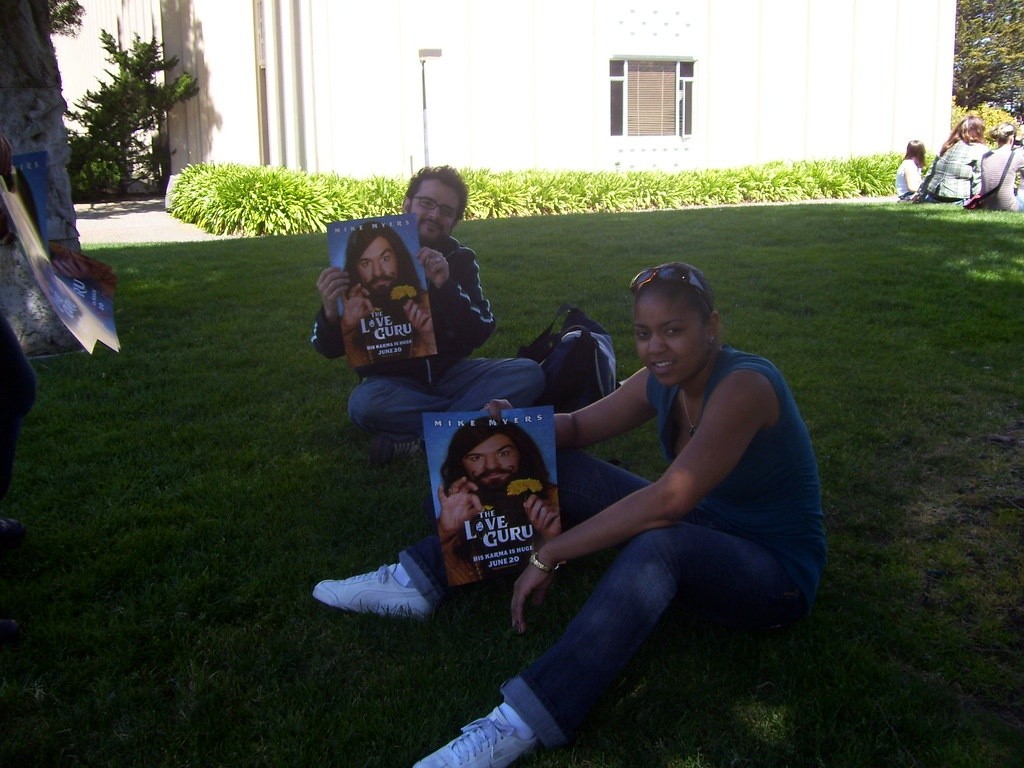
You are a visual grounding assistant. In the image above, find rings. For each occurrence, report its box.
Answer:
[436,257,440,262]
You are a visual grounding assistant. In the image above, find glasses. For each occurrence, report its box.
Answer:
[412,195,459,220]
[629,264,713,314]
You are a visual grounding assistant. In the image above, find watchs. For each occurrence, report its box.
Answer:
[528,550,568,573]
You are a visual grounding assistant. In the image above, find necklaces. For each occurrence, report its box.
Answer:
[683,390,701,436]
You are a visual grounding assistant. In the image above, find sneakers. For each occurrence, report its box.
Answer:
[413,706,537,768]
[370,430,425,464]
[312,563,431,619]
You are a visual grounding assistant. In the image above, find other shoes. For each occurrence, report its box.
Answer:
[0,619,19,640]
[0,518,26,551]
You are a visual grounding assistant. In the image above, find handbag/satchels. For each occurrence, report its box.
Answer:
[516,302,616,414]
[963,193,982,210]
[911,156,939,204]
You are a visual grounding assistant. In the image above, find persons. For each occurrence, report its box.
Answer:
[312,262,832,768]
[435,416,562,588]
[0,314,38,642]
[895,139,927,201]
[927,117,1024,212]
[341,222,437,368]
[312,165,545,464]
